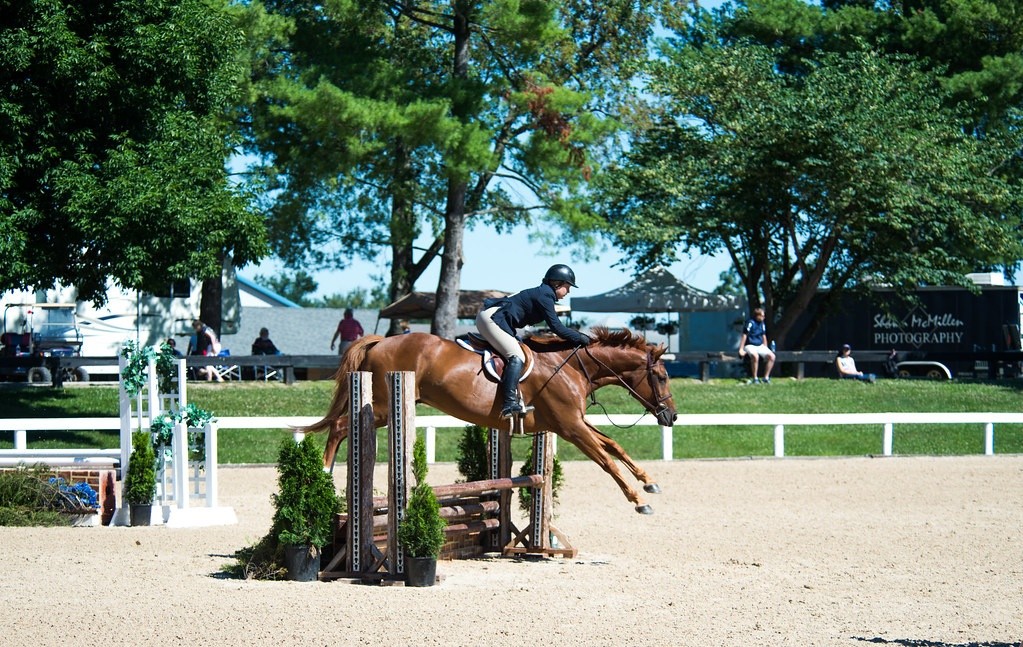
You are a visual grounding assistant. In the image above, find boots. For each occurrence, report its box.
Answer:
[499,356,525,414]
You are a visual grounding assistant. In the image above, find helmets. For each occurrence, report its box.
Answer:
[545,264,579,289]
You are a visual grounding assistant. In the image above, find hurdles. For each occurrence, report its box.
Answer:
[318,370,577,584]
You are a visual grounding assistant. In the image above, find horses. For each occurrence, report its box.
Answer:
[281,324,680,516]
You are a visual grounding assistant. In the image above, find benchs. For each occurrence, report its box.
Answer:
[51,346,932,384]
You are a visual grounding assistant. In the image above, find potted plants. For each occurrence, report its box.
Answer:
[396,436,442,589]
[124,428,158,525]
[271,436,340,583]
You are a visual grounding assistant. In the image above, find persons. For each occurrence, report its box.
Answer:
[167,339,182,355]
[738,308,775,385]
[187,333,199,354]
[331,309,364,356]
[475,264,593,421]
[252,328,281,354]
[400,322,410,333]
[192,320,227,383]
[836,344,875,382]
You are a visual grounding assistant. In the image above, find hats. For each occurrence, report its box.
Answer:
[843,344,851,350]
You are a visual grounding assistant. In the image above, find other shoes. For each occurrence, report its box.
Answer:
[763,377,772,383]
[754,378,762,384]
